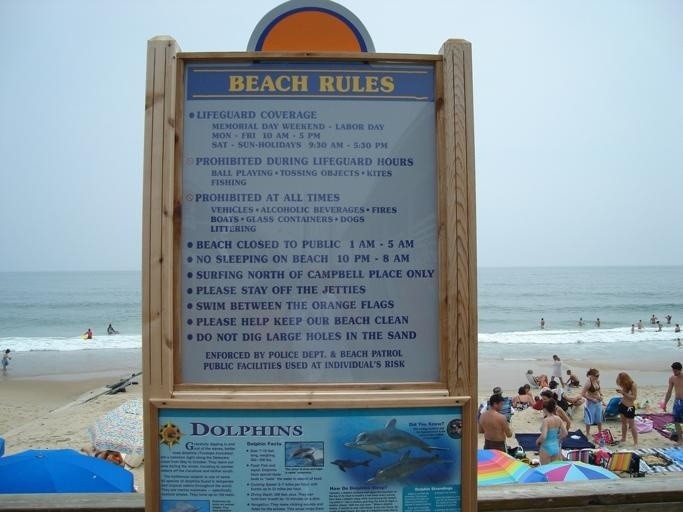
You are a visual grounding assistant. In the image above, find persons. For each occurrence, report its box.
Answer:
[84,328,92,339]
[1,349,12,376]
[108,324,115,335]
[478,314,683,465]
[81,441,122,464]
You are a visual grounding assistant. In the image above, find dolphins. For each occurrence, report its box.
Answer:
[330,458,372,472]
[344,418,451,458]
[287,445,316,467]
[366,449,456,485]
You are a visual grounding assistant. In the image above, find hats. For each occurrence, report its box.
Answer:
[492,386,503,396]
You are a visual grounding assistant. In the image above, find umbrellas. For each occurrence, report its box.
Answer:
[477,449,621,485]
[0,448,135,493]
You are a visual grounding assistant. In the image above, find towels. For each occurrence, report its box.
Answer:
[632,446,683,474]
[636,412,674,439]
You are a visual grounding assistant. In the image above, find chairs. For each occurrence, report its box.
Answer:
[486,397,513,425]
[605,395,623,420]
[525,372,539,387]
[599,450,635,478]
[565,448,594,464]
